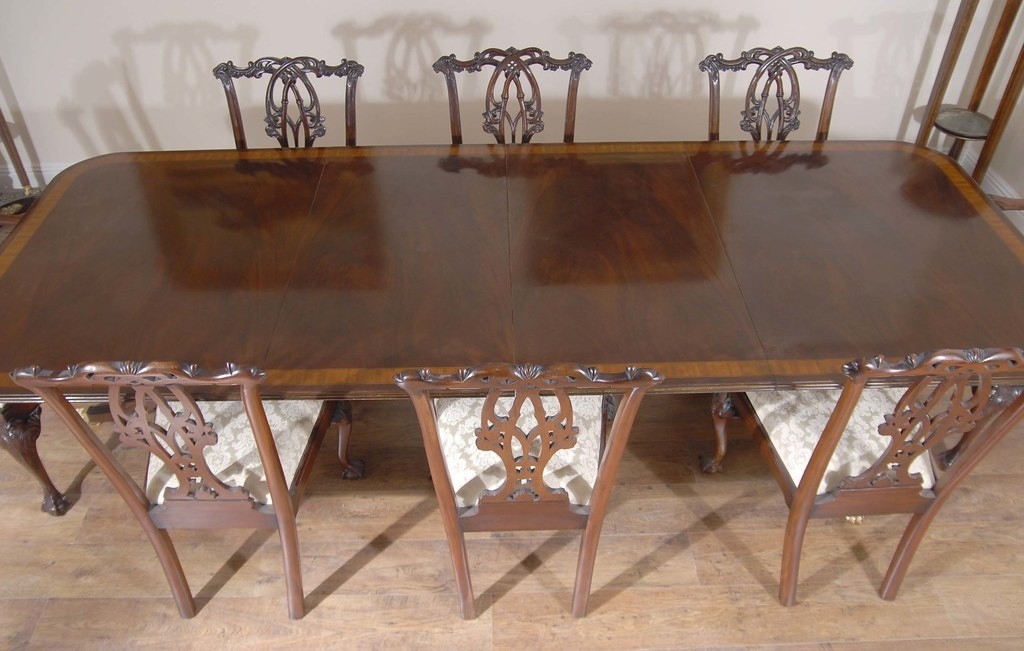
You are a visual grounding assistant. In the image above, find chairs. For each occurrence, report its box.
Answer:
[700,345,1023,608]
[212,55,364,150]
[699,47,854,141]
[0,109,71,516]
[10,356,365,621]
[433,46,592,145]
[394,361,666,621]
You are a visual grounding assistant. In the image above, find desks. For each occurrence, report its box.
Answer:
[0,138,1024,516]
